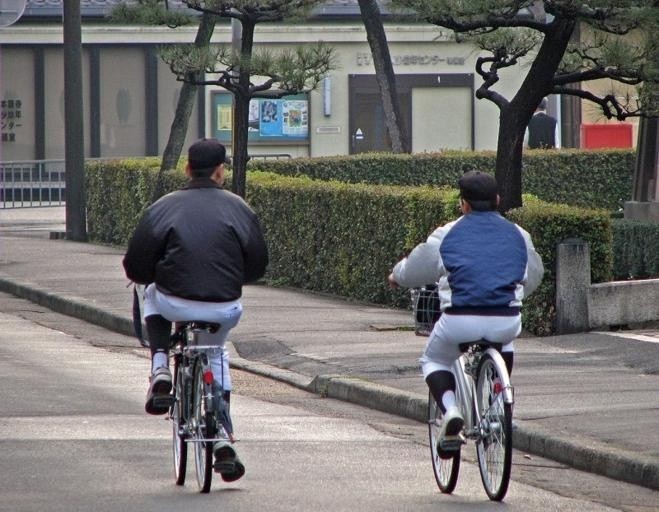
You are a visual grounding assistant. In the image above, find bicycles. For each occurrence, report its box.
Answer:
[124,281,239,494]
[394,278,516,502]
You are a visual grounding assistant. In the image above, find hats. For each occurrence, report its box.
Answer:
[187,137,232,165]
[457,169,501,202]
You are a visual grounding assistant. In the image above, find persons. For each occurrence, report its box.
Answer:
[121,137,271,484]
[387,169,546,461]
[521,95,562,149]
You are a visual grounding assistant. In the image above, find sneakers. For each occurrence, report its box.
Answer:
[213,437,246,482]
[436,408,465,460]
[144,364,173,415]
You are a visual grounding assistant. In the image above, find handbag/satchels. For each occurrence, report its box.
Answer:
[414,282,441,322]
